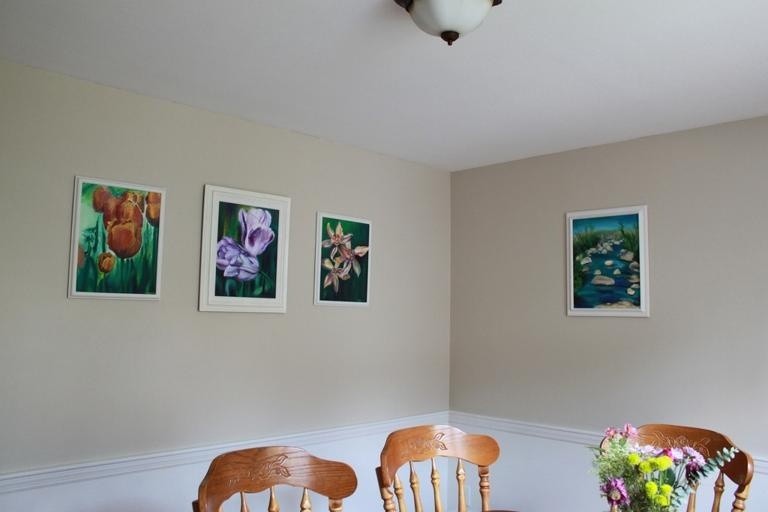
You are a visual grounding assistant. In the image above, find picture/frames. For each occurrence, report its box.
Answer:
[196,183,294,315]
[564,204,651,318]
[313,209,374,308]
[65,172,167,301]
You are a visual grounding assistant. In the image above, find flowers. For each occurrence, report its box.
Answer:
[77,186,160,280]
[320,220,370,297]
[592,422,740,511]
[214,205,277,299]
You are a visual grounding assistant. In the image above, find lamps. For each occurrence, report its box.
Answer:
[396,0,504,46]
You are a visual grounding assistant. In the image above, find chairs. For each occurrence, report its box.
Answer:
[370,421,501,512]
[631,423,756,511]
[189,439,360,511]
